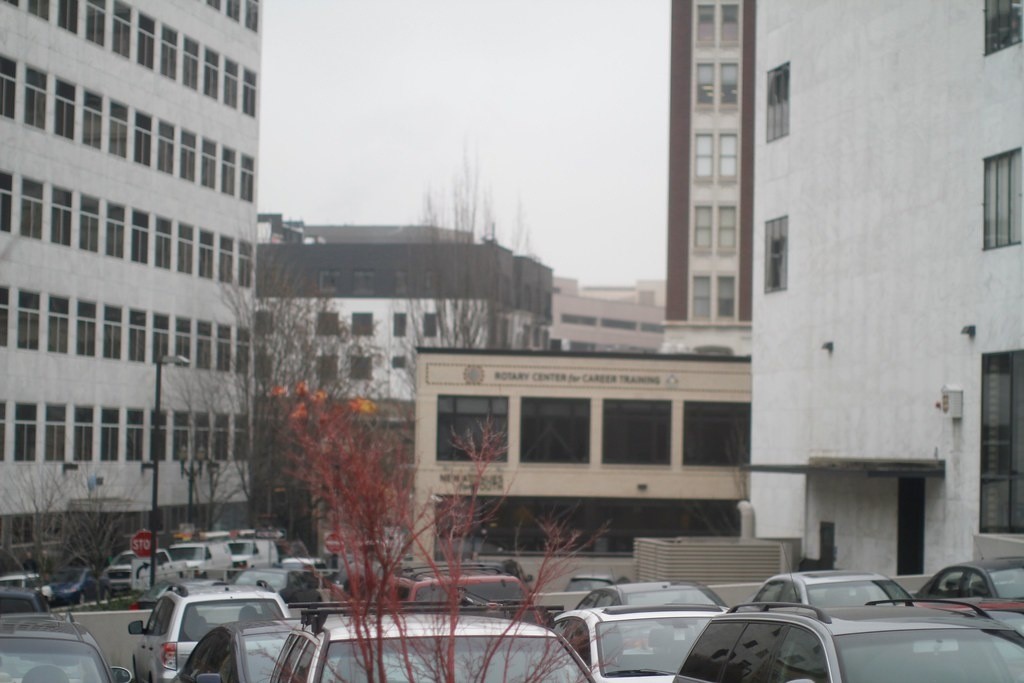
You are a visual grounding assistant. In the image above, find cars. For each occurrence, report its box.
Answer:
[0,590,50,614]
[170,555,1024,683]
[0,615,134,683]
[36,567,112,607]
[0,571,46,591]
[128,585,292,683]
[99,528,289,596]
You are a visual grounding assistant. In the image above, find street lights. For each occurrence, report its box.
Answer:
[150,353,190,592]
